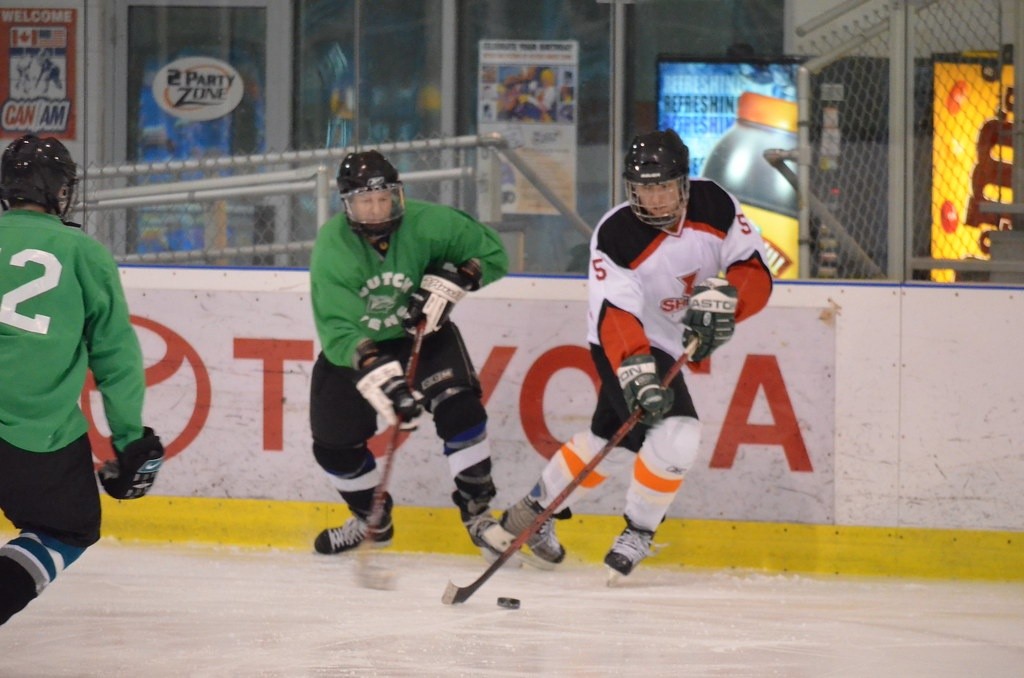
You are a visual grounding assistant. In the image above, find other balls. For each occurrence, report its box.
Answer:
[497,594,523,612]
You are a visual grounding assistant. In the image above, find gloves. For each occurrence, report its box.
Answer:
[352,352,426,432]
[400,261,475,336]
[679,275,740,363]
[616,354,675,425]
[96,426,166,501]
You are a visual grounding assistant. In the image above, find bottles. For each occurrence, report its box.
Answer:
[704,92,799,280]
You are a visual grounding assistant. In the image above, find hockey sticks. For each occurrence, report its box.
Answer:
[439,335,703,607]
[345,317,438,591]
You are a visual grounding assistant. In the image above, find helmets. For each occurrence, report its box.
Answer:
[0,133,79,203]
[621,128,690,184]
[336,150,405,199]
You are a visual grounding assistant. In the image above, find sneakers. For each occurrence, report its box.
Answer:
[604,513,668,588]
[451,491,523,570]
[500,476,573,565]
[313,509,395,555]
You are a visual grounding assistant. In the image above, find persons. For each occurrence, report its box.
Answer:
[0,135,167,624]
[306,150,526,568]
[500,128,773,588]
[479,61,575,124]
